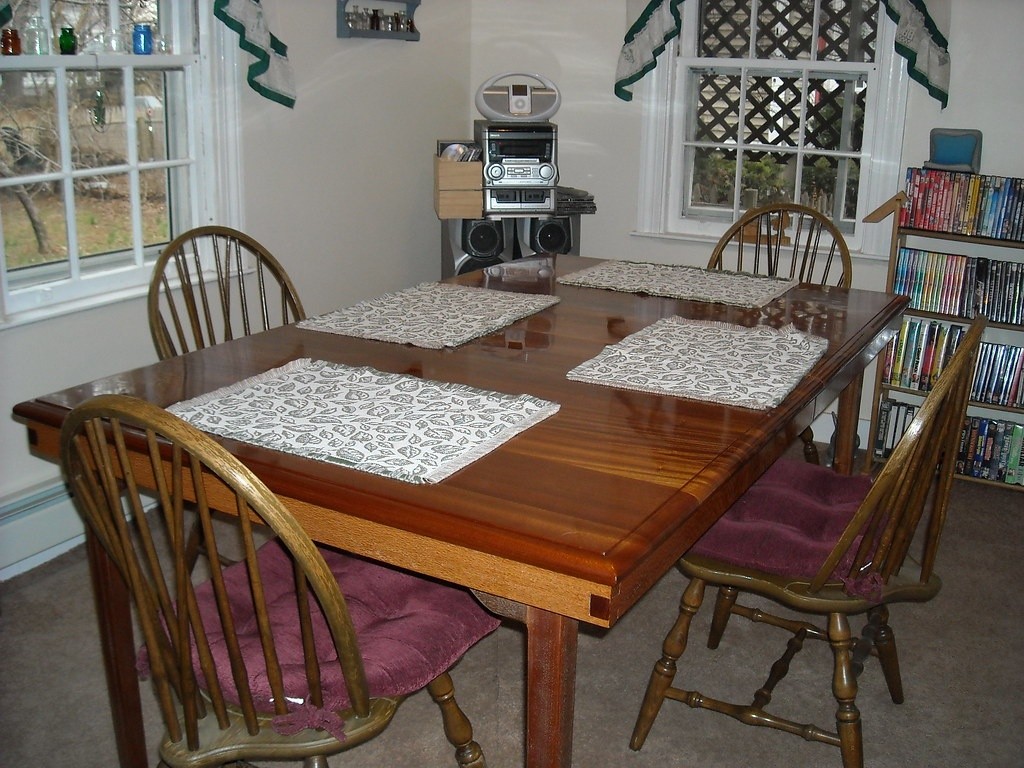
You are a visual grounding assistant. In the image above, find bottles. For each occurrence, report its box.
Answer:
[23,16,49,55]
[59,28,78,55]
[347,5,413,32]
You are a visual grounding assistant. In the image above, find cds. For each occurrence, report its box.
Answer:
[440,144,469,161]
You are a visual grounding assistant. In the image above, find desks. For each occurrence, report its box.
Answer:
[12,250,909,768]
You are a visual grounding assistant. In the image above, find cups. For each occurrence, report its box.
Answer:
[132,24,153,55]
[156,35,173,54]
[1,29,22,55]
[104,28,127,54]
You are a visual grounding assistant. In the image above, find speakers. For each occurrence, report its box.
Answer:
[514,215,581,260]
[441,218,514,279]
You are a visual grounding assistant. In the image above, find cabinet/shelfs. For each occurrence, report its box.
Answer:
[861,190,1024,493]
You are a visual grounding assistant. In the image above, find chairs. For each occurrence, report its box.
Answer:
[147,226,308,361]
[60,393,501,768]
[626,314,990,768]
[706,203,852,291]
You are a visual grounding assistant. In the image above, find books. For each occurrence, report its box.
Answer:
[874,399,1023,486]
[894,247,1024,325]
[900,167,1024,241]
[883,317,1023,409]
